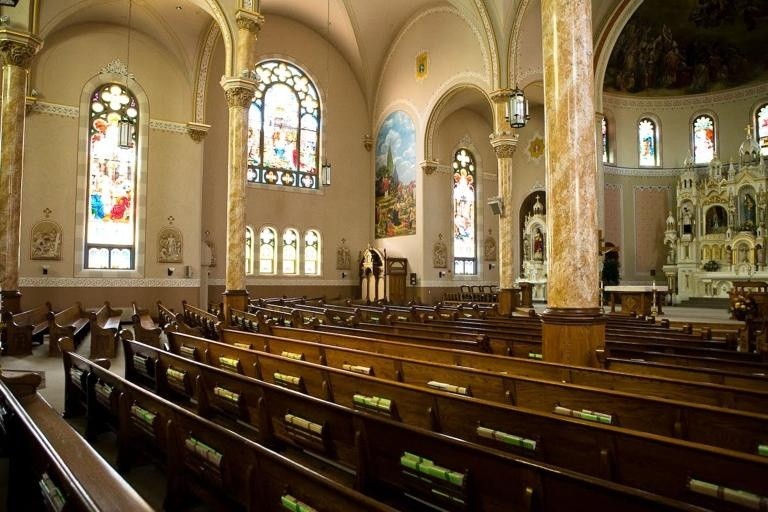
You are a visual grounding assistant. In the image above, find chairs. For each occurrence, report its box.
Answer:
[460,285,498,301]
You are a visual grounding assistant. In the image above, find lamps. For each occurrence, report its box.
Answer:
[118,1,134,149]
[505,88,530,128]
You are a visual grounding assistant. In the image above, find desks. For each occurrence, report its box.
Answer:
[605,286,668,315]
